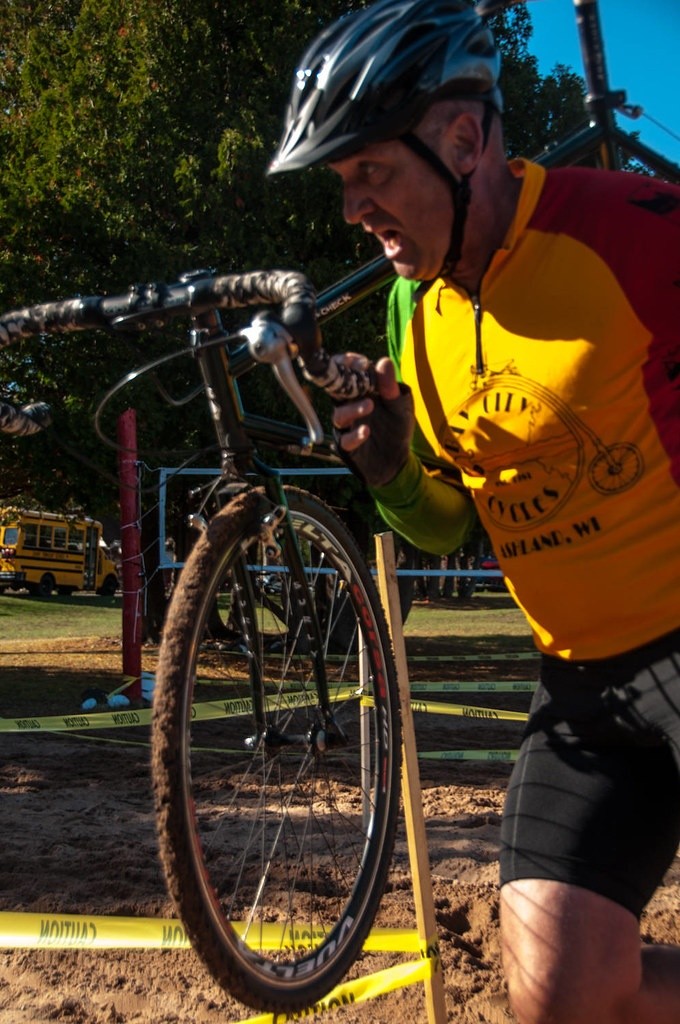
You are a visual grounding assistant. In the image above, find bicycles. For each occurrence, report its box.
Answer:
[0,0,679,1008]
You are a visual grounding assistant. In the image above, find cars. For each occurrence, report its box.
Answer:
[265,579,314,596]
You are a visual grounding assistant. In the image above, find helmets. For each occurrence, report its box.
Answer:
[264,0,500,177]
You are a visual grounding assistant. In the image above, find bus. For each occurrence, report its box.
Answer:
[0,506,121,599]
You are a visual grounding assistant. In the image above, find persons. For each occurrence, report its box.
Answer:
[265,0,680,1024]
